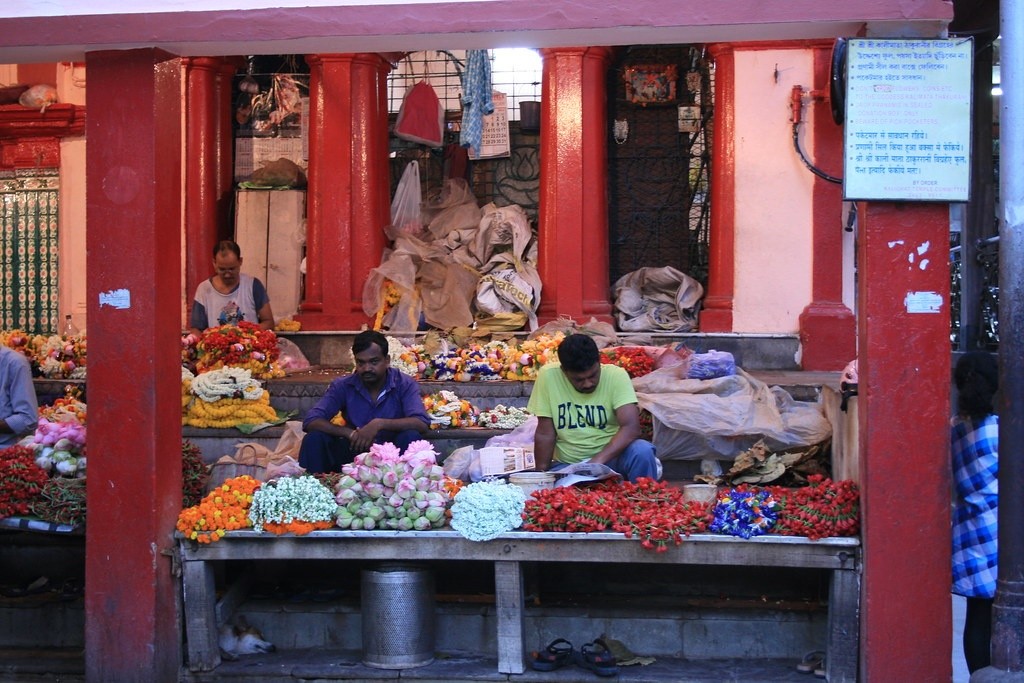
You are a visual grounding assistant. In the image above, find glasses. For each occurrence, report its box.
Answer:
[215,262,240,275]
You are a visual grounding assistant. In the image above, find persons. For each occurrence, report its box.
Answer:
[0,344,39,450]
[191,241,274,338]
[298,330,431,475]
[949,351,1001,676]
[526,333,656,488]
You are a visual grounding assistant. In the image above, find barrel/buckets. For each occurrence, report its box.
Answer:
[683,483,718,513]
[359,570,434,668]
[508,472,555,499]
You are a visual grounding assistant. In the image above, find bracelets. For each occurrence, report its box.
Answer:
[349,430,357,441]
